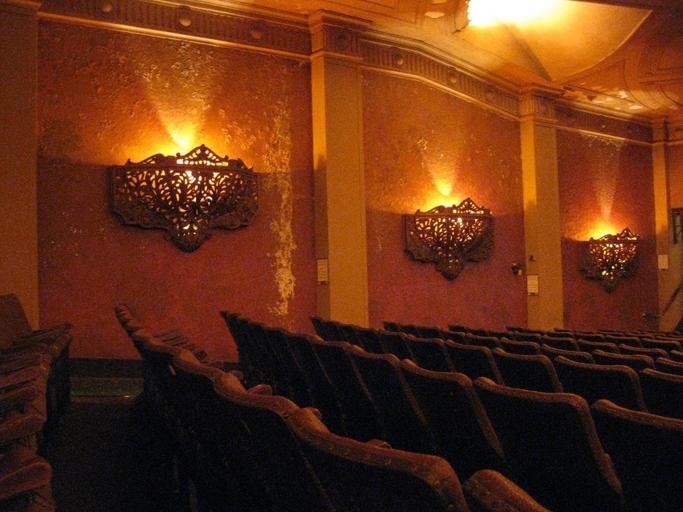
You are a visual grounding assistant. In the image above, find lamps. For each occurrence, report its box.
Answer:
[103,138,257,256]
[576,222,639,290]
[398,194,493,281]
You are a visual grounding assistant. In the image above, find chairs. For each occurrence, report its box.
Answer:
[0,292,682,512]
